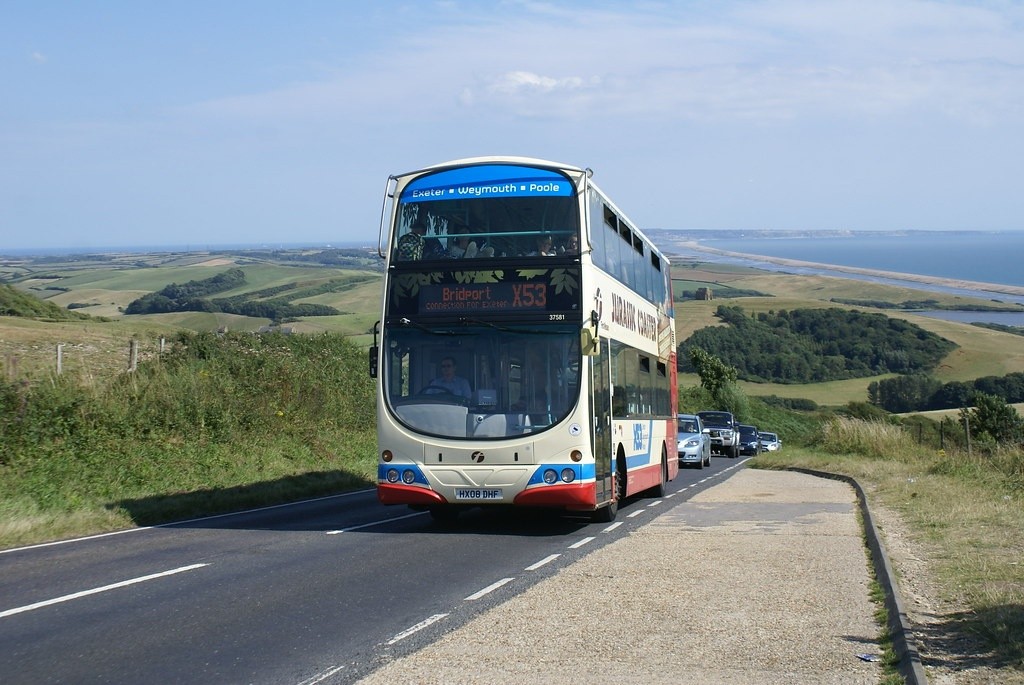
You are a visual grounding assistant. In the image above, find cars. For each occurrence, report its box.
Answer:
[677,414,712,467]
[758,432,783,452]
[739,425,762,456]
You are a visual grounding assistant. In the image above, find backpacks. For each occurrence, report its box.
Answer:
[396,232,425,262]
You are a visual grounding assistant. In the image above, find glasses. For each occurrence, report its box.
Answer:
[440,364,453,368]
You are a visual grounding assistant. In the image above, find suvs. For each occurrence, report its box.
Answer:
[697,410,741,458]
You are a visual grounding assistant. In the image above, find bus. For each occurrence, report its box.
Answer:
[370,154,679,522]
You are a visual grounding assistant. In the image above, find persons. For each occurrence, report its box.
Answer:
[685,423,694,433]
[424,357,472,406]
[407,222,580,258]
[597,249,630,285]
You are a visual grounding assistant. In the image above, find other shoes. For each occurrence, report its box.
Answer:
[464,242,477,258]
[477,247,495,258]
